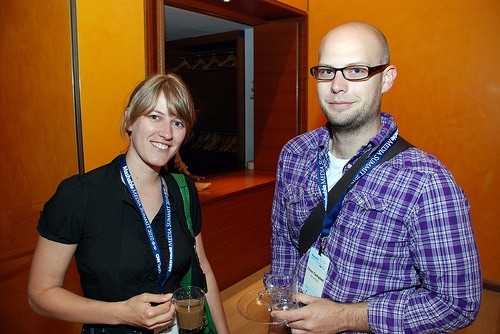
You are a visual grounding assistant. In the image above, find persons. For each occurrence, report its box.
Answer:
[27,73,231,334]
[167,151,205,182]
[262,21,483,334]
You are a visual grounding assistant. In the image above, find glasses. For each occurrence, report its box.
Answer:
[310,64,390,81]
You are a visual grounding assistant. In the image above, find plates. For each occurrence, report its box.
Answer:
[236,286,294,325]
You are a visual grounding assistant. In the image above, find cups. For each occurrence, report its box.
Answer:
[259,274,299,320]
[169,285,205,334]
